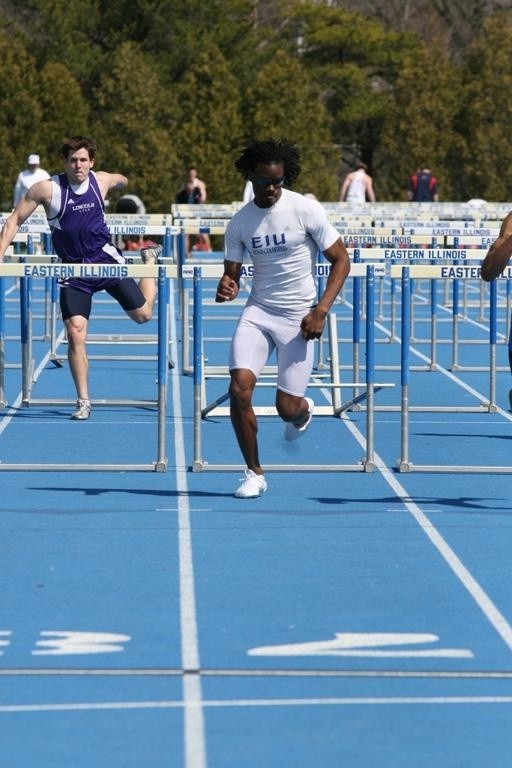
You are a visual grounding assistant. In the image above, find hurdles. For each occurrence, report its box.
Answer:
[0,201,278,375]
[313,202,511,346]
[389,263,511,473]
[2,254,174,407]
[170,226,281,375]
[341,247,498,414]
[445,234,511,372]
[181,261,387,474]
[317,234,445,374]
[0,263,179,472]
[200,312,397,420]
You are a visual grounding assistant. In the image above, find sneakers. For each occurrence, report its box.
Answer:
[139,244,164,263]
[281,396,315,443]
[67,398,94,421]
[231,465,272,500]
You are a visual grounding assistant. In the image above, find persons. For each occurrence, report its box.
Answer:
[479,209,512,284]
[173,177,203,260]
[337,160,376,203]
[10,151,54,213]
[184,167,213,254]
[406,158,440,202]
[1,132,165,421]
[114,192,147,252]
[213,138,351,499]
